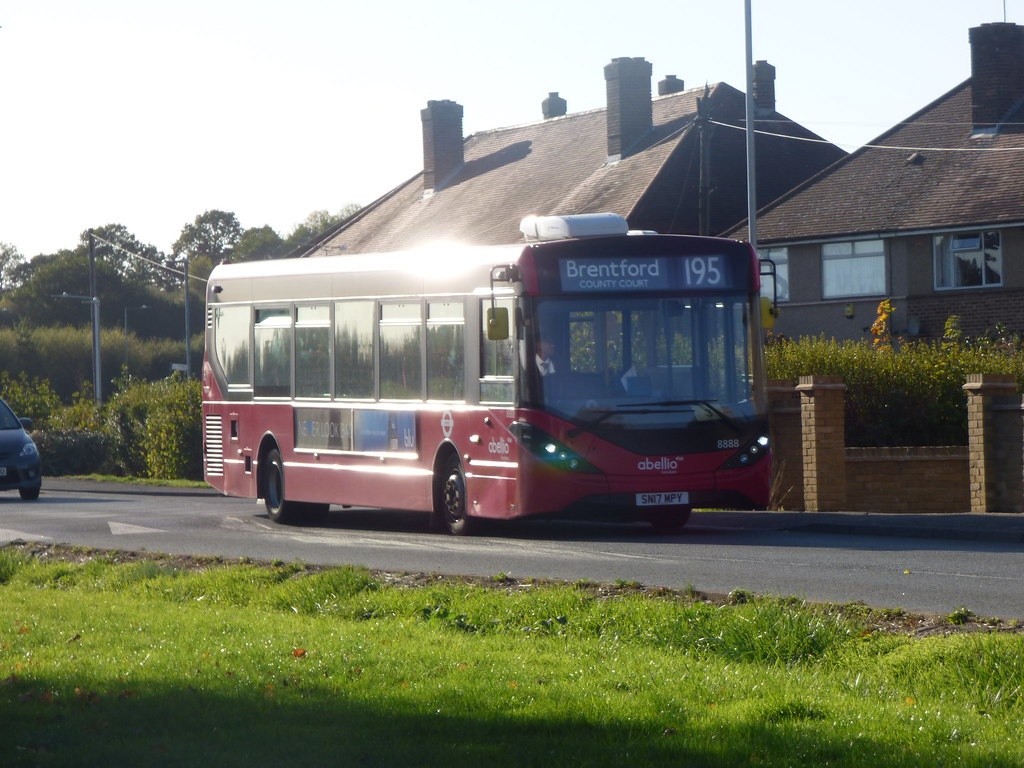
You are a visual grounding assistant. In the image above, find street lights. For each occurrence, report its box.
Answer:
[50,294,101,404]
[123,305,148,393]
[164,259,190,382]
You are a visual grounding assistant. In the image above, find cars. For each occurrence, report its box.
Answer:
[0,397,42,500]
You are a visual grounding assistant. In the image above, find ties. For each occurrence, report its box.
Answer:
[540,361,550,375]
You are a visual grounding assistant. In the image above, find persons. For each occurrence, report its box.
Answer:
[533,332,556,377]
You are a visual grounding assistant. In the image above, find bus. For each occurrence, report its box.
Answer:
[198,210,779,539]
